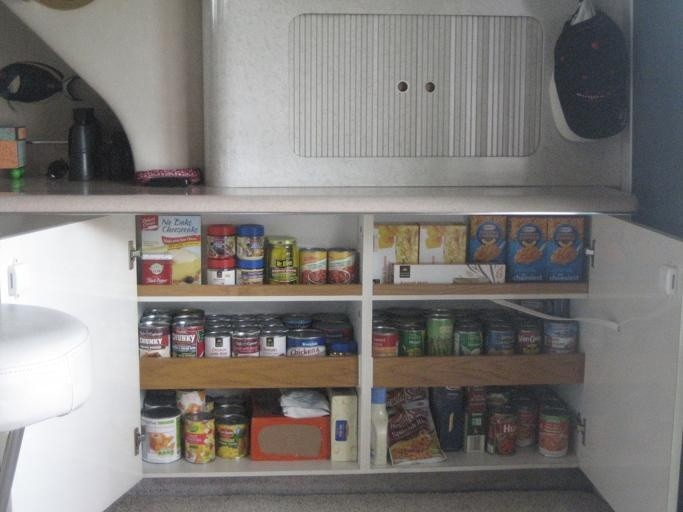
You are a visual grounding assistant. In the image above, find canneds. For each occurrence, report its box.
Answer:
[206,222,356,284]
[486,385,571,458]
[141,389,249,464]
[372,306,579,358]
[139,306,354,359]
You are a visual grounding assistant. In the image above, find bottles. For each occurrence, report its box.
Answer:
[372,387,390,468]
[67,108,96,181]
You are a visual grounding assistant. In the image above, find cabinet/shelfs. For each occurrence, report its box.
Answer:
[133,214,628,491]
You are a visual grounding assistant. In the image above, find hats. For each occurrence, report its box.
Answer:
[550,0,630,142]
